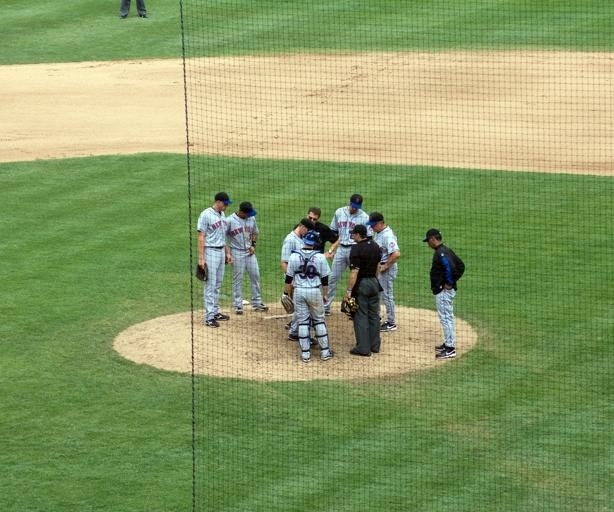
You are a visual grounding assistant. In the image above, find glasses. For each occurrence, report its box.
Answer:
[309,216,317,220]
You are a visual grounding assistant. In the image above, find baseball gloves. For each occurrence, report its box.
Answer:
[196,264,209,281]
[341,297,359,318]
[281,295,293,313]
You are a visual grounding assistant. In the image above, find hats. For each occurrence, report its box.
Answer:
[366,213,383,225]
[240,201,256,215]
[351,194,363,209]
[349,225,367,234]
[215,192,233,204]
[301,218,315,230]
[422,229,439,242]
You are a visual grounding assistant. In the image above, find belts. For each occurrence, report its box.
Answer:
[341,244,352,248]
[210,245,224,250]
[380,263,385,265]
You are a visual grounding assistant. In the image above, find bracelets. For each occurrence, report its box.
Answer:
[346,288,353,292]
[283,291,288,295]
[251,240,257,248]
[328,248,333,254]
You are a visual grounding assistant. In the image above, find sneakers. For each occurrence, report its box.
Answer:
[380,323,396,332]
[236,307,242,313]
[302,351,333,361]
[215,313,229,321]
[253,303,269,312]
[350,349,371,357]
[436,343,456,358]
[120,13,146,18]
[207,320,219,327]
[325,311,330,316]
[285,321,318,345]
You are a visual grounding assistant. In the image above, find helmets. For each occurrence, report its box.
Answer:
[303,230,321,245]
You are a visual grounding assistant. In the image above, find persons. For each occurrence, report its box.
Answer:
[422,227,465,359]
[118,0,150,20]
[364,212,399,333]
[280,229,334,364]
[281,218,319,347]
[195,191,233,330]
[224,201,269,314]
[344,225,382,358]
[292,205,342,259]
[323,193,374,317]
